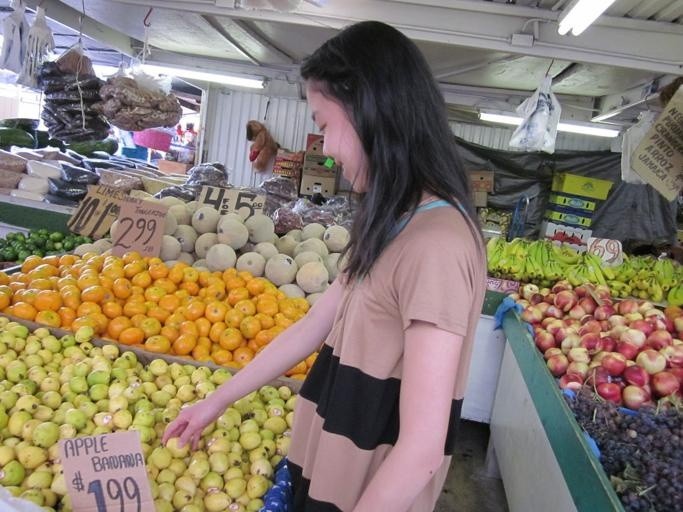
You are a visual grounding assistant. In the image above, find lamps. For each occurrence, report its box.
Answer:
[557,0,616,37]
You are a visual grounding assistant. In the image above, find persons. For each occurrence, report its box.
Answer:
[162,21,488,512]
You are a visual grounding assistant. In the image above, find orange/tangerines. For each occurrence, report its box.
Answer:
[0,251,326,381]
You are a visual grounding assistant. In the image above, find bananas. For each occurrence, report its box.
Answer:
[486,235,683,305]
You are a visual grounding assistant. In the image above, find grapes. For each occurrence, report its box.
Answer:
[565,385,683,511]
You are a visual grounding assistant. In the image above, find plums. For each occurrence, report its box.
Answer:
[0,316,298,512]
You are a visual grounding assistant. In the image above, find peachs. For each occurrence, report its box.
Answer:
[506,279,682,408]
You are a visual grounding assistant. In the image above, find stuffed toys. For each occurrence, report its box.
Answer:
[246,120,280,172]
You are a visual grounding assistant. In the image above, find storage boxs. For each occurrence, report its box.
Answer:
[300,174,340,198]
[274,158,301,170]
[276,146,304,163]
[303,153,341,179]
[305,133,325,155]
[675,228,683,247]
[538,219,594,246]
[473,193,489,209]
[541,205,593,229]
[546,191,599,215]
[466,168,495,193]
[272,166,300,178]
[551,171,615,201]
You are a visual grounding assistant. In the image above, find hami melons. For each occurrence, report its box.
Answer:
[73,195,352,303]
